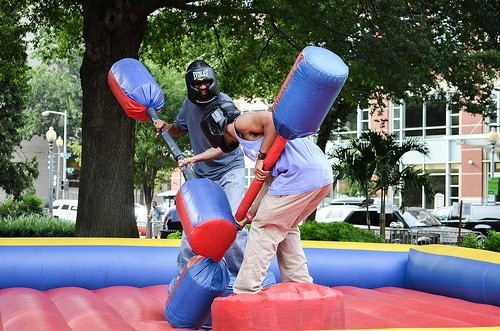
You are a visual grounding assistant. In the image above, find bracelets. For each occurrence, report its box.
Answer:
[257,150,267,160]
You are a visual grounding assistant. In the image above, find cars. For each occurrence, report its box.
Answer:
[133,202,147,235]
[314,196,500,245]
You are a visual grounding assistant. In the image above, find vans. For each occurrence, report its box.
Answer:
[47,199,78,223]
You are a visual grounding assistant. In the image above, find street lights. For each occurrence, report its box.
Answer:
[46,126,56,216]
[41,109,67,199]
[487,126,498,178]
[55,135,63,200]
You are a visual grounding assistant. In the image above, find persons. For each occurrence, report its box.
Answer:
[149,200,161,239]
[162,197,185,234]
[204,99,336,296]
[152,60,276,279]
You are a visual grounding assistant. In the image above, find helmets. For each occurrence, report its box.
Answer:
[184,60,220,105]
[200,99,239,153]
